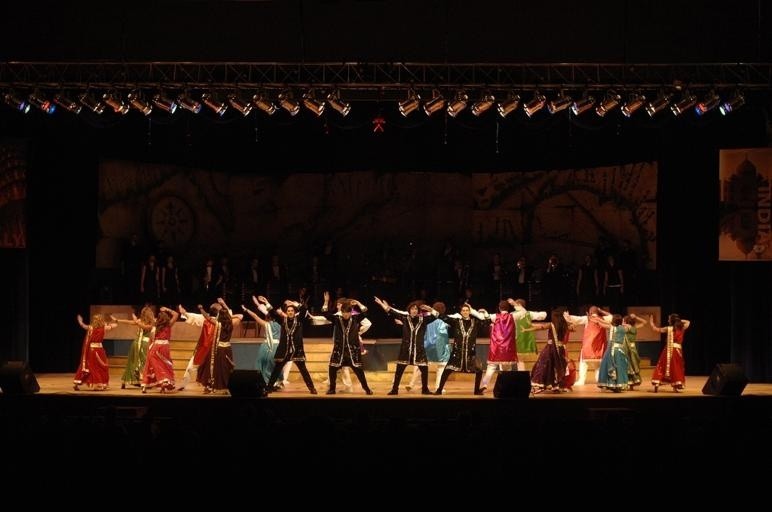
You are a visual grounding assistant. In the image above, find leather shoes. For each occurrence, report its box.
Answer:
[367,390,373,394]
[328,389,335,394]
[474,387,486,394]
[433,390,442,395]
[311,388,317,394]
[422,389,433,394]
[388,390,398,395]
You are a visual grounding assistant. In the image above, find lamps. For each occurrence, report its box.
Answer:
[700,362,753,399]
[0,59,353,121]
[397,62,748,119]
[225,369,270,399]
[0,358,41,396]
[494,370,535,399]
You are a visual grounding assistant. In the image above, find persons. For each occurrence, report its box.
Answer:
[76,313,119,392]
[243,292,376,396]
[374,297,572,395]
[111,297,243,393]
[125,233,623,312]
[563,308,691,396]
